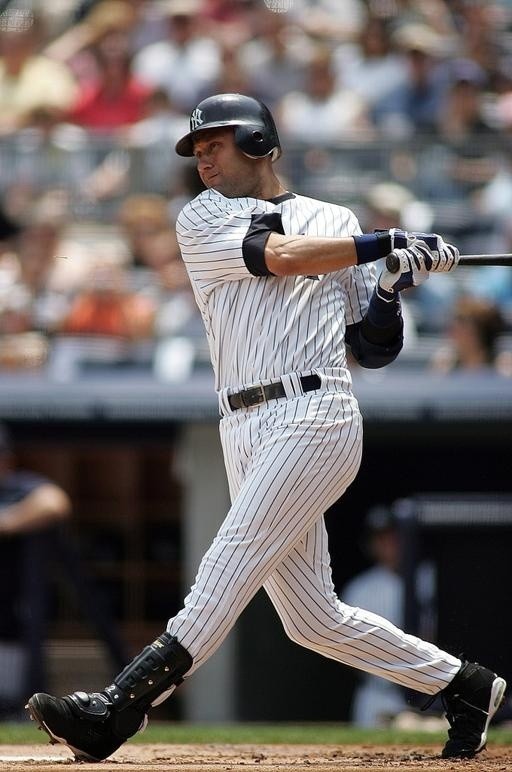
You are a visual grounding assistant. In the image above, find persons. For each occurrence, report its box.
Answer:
[20,90,509,769]
[0,0,510,378]
[342,500,440,730]
[1,445,74,723]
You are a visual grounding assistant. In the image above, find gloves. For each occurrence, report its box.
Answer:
[373,228,460,299]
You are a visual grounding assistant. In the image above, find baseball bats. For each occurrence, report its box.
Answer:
[386,253,512,272]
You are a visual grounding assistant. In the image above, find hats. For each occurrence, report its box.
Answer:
[176,93,281,163]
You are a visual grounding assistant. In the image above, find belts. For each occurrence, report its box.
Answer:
[228,374,321,411]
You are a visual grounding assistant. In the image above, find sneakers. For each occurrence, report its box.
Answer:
[25,691,136,761]
[421,652,506,758]
